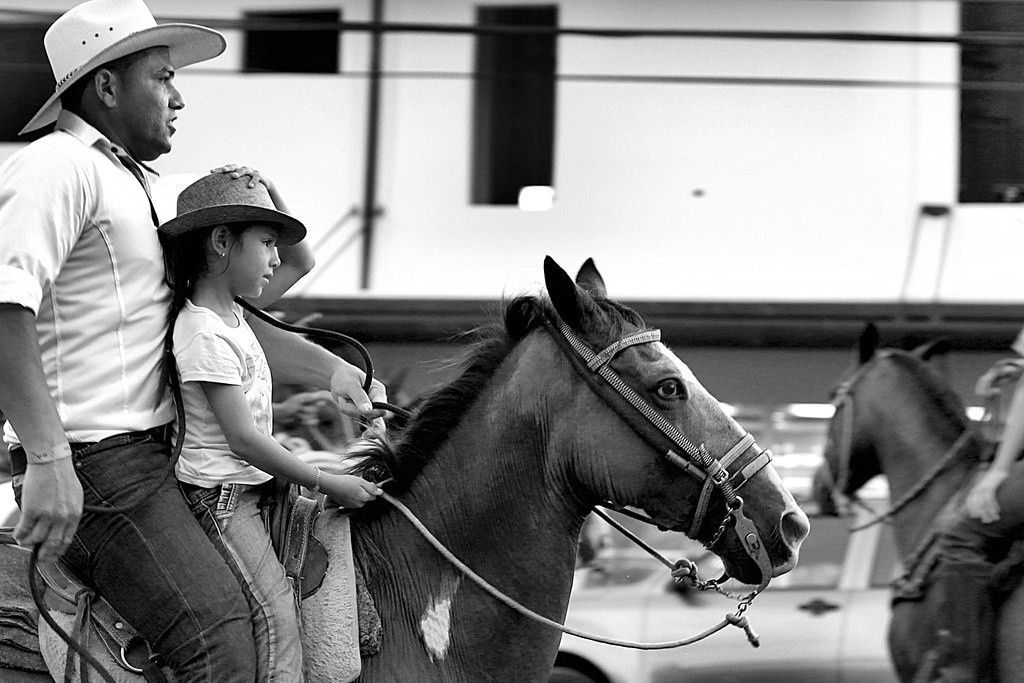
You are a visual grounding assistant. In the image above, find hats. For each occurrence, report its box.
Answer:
[17,0,227,135]
[156,172,306,246]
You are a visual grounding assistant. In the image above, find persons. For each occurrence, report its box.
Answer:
[0,0,258,682]
[157,164,382,682]
[966,322,1024,523]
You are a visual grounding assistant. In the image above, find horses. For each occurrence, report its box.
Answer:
[0,253,813,683]
[813,320,1024,683]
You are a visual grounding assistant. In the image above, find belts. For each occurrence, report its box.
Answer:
[9,421,176,477]
